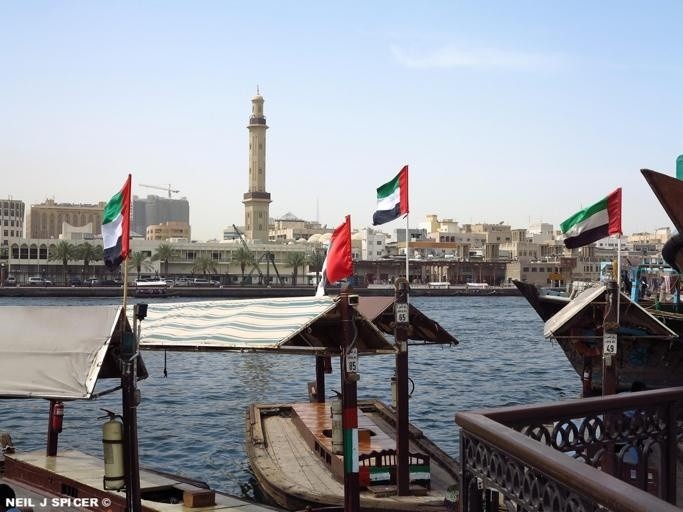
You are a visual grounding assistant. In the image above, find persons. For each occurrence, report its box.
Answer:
[622,267,666,297]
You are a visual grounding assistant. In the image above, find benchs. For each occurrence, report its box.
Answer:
[373,165,409,227]
[559,187,623,250]
[325,215,353,285]
[101,175,129,272]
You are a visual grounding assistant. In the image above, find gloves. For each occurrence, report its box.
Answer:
[511,154,683,389]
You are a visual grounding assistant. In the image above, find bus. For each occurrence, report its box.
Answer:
[466,283,488,289]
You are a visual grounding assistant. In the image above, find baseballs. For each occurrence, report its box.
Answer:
[328,389,344,455]
[97,408,126,492]
[324,357,332,374]
[391,370,414,409]
[52,400,64,433]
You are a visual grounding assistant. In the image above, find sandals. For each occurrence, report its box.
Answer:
[466,283,488,289]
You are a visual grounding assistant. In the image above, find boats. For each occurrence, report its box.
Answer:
[0,164,461,512]
[511,154,683,389]
